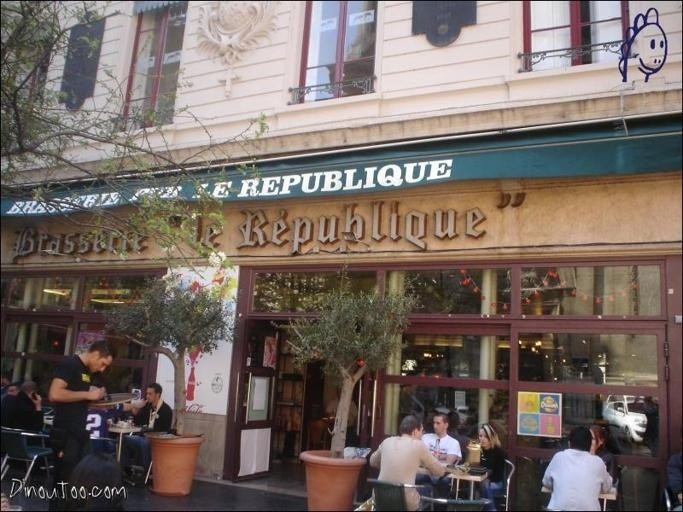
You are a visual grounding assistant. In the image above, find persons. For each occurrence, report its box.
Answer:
[369,413,508,512]
[642,396,658,454]
[399,373,425,426]
[594,394,603,419]
[0,339,173,512]
[541,424,624,511]
[667,451,683,512]
[325,386,358,432]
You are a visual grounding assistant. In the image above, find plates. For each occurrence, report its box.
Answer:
[468,467,488,476]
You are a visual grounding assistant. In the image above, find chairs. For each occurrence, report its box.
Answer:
[0,424,54,500]
[499,461,518,511]
[424,496,492,512]
[660,489,675,511]
[363,479,434,512]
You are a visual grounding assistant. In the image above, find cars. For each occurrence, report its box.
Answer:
[602,394,648,443]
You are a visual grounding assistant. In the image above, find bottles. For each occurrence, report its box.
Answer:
[127,417,134,429]
[434,440,441,460]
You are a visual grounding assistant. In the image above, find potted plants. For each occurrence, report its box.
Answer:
[102,264,238,497]
[269,257,421,511]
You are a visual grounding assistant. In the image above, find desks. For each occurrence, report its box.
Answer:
[539,481,619,512]
[108,426,142,463]
[423,463,492,512]
